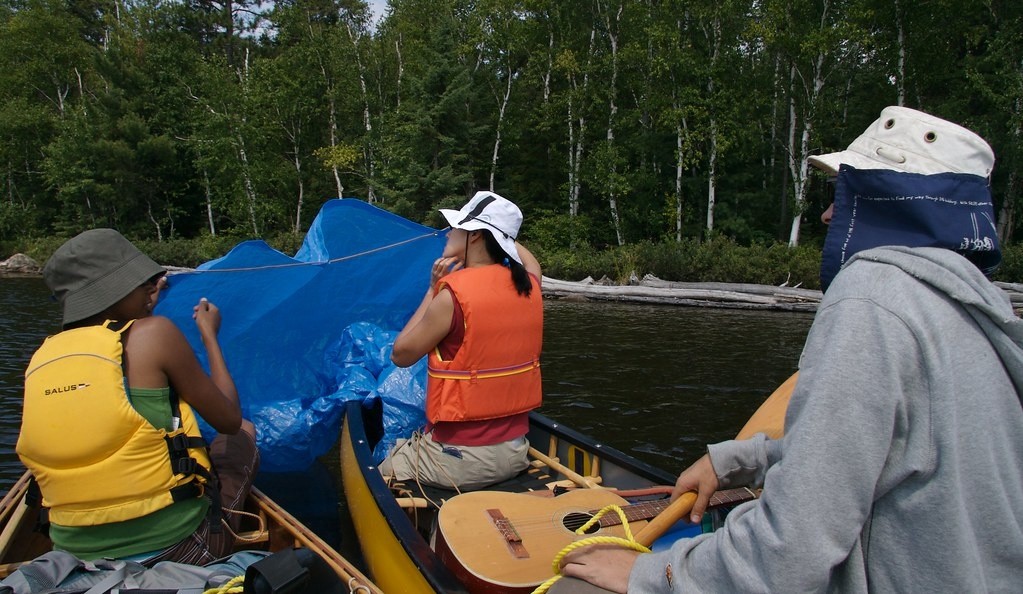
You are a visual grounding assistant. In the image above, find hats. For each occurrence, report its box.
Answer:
[43,228,167,332]
[438,191,523,265]
[807,106,995,179]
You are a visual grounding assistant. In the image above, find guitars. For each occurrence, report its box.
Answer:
[435,485,764,594]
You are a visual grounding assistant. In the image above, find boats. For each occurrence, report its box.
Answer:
[341,387,737,594]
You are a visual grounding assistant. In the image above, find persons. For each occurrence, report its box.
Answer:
[375,189,544,490]
[22,227,258,565]
[549,103,1021,593]
